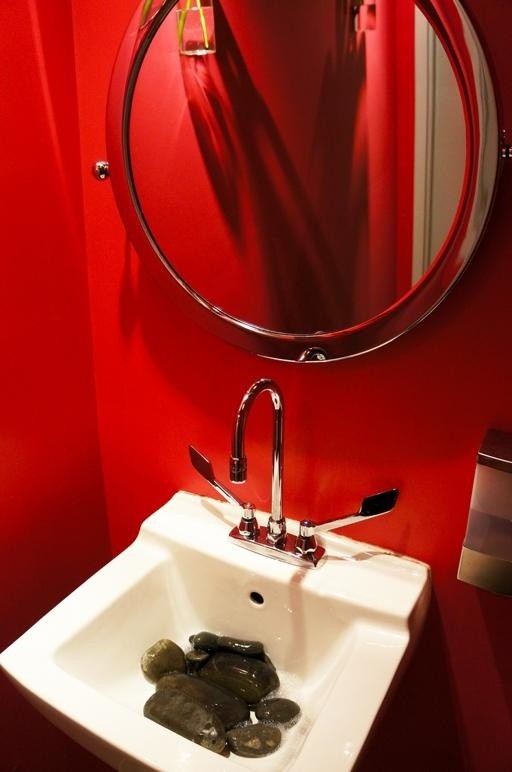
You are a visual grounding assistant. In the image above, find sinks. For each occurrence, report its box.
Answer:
[0,491,432,772]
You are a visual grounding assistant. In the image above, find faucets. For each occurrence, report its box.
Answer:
[230,377,284,544]
[296,344,331,365]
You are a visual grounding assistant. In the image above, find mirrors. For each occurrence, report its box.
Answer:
[103,0,510,366]
